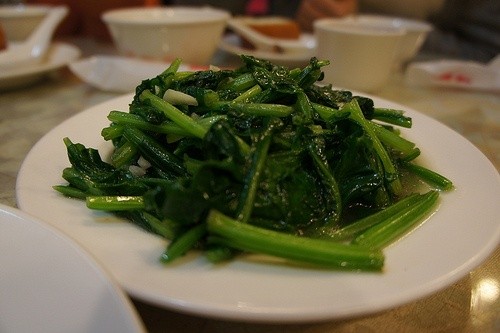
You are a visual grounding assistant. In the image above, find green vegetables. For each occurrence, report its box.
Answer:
[53,54,454,273]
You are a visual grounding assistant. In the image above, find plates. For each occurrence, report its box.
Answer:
[221,30,318,70]
[0,39,81,93]
[65,55,197,93]
[15,84,500,322]
[0,201,145,333]
[424,58,500,93]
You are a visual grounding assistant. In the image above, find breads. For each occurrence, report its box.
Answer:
[237,18,300,52]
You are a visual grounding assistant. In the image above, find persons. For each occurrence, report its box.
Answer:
[280,0,500,65]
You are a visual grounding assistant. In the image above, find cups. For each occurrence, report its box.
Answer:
[102,8,228,68]
[347,13,434,73]
[0,7,70,70]
[313,17,407,94]
